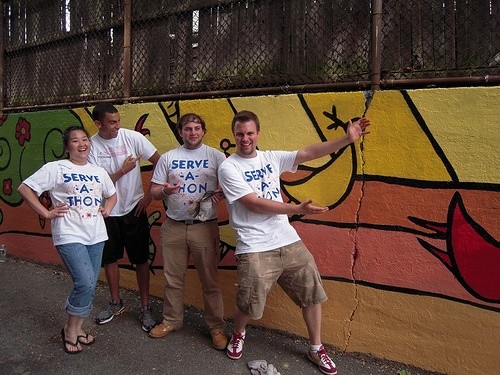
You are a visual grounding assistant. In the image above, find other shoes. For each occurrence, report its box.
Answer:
[148,318,184,339]
[209,331,229,350]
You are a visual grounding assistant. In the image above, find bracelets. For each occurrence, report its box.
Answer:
[162,188,169,197]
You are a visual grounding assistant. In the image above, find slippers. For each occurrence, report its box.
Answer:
[61,328,95,355]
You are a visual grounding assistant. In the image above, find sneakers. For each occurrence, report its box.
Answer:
[227,329,246,360]
[96,299,125,326]
[137,304,158,332]
[306,346,338,375]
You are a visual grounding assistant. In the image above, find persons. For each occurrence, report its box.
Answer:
[217,110,371,375]
[149,113,228,350]
[17,127,118,354]
[86,101,162,332]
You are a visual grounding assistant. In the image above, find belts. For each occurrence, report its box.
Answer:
[166,214,218,225]
